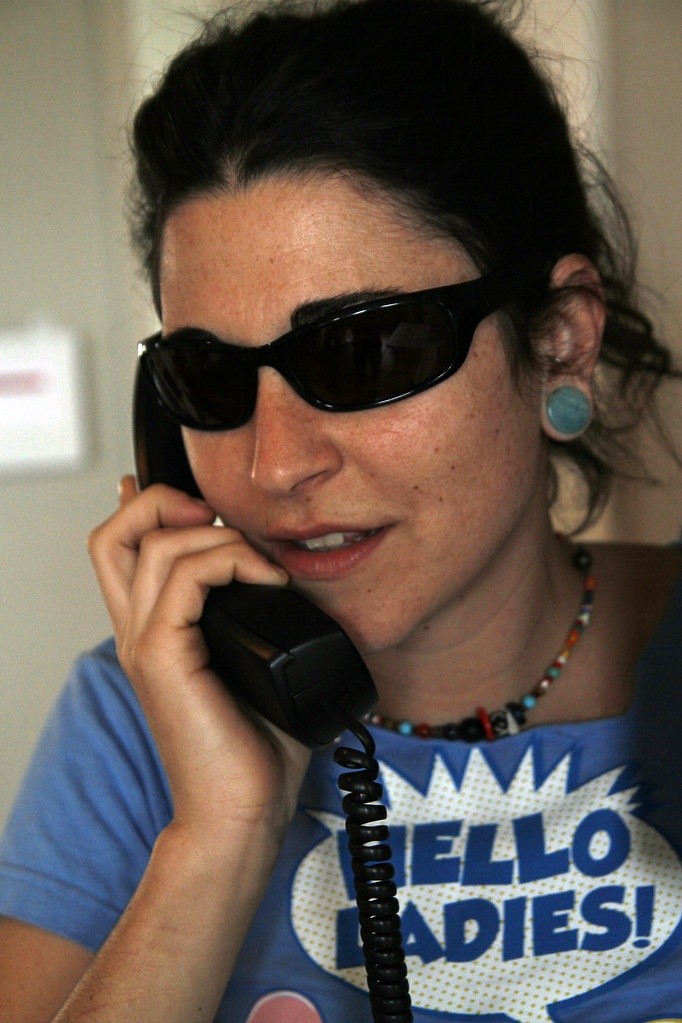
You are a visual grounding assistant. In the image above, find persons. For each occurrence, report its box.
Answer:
[1,0,682,1023]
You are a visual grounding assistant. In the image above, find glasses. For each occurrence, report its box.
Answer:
[136,268,517,431]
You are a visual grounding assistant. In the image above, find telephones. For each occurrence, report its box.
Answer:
[132,333,381,754]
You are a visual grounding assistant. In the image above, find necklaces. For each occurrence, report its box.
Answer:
[361,531,597,740]
